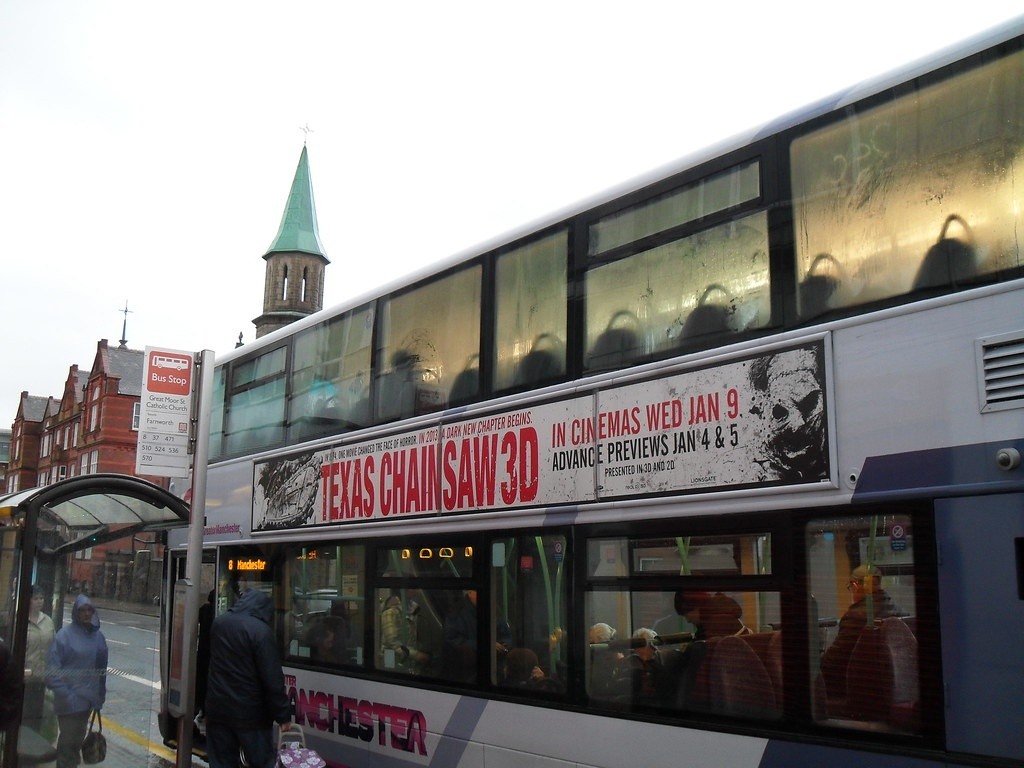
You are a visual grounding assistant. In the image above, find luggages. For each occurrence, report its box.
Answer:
[276,723,326,768]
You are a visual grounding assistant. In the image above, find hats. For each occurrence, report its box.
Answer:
[506,648,538,678]
[674,592,711,615]
[701,596,742,635]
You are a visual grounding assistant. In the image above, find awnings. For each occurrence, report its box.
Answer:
[0,472,205,564]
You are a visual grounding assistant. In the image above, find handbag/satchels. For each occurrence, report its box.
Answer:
[81,709,106,764]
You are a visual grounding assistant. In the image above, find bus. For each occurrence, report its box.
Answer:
[154,10,1024,768]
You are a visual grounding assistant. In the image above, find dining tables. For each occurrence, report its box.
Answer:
[707,636,777,716]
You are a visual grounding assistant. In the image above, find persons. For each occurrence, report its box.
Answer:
[382,351,420,425]
[197,565,910,768]
[21,585,108,768]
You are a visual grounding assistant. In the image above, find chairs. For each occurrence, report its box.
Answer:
[446,353,479,402]
[586,310,647,371]
[677,283,743,346]
[765,251,849,328]
[309,614,351,663]
[605,655,645,707]
[319,396,351,419]
[511,332,566,386]
[911,213,976,292]
[392,368,447,416]
[766,630,830,721]
[880,616,919,724]
[841,624,877,701]
[347,386,375,426]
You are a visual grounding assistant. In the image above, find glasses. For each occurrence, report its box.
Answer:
[846,585,856,591]
